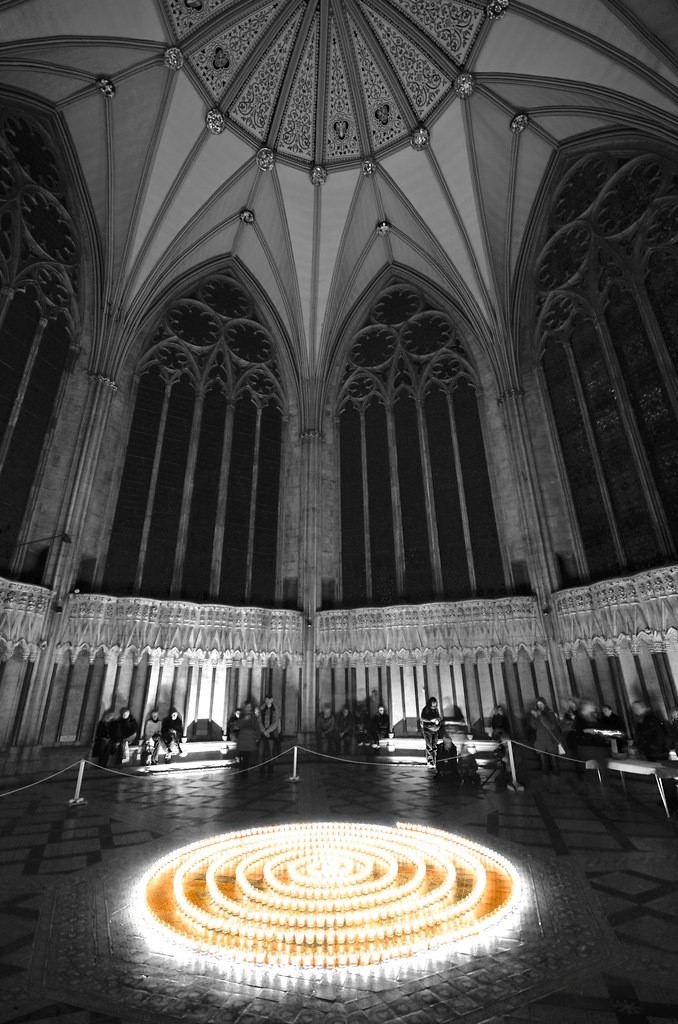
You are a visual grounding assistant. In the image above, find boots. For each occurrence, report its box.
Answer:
[542,774,550,790]
[549,775,561,793]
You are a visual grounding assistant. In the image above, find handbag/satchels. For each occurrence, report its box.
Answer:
[558,742,566,755]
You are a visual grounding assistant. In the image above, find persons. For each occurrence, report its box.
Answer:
[316,704,390,756]
[144,710,186,766]
[229,695,279,775]
[491,707,511,762]
[92,709,138,769]
[421,697,454,780]
[629,700,678,818]
[527,696,626,782]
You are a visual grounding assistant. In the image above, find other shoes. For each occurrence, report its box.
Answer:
[372,744,380,749]
[427,763,436,769]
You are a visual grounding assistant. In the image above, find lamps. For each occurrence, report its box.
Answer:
[16,533,72,547]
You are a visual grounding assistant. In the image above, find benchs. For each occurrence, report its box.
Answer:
[378,733,501,755]
[129,736,237,760]
